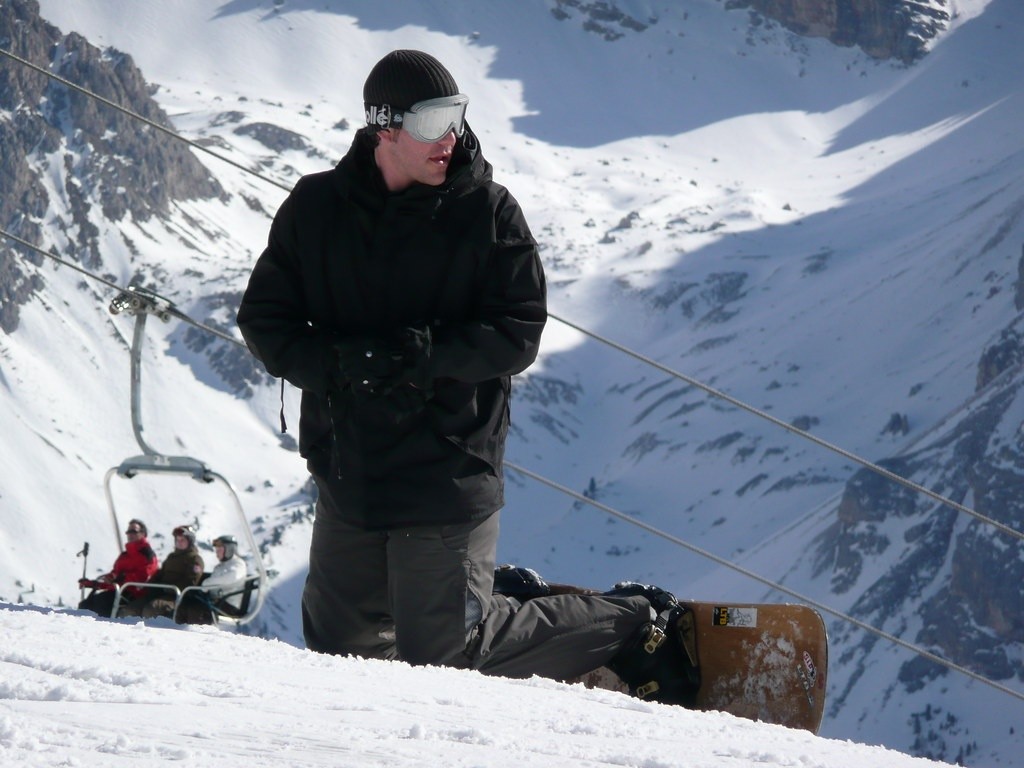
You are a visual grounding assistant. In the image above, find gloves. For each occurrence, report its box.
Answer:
[337,330,434,414]
[80,579,94,589]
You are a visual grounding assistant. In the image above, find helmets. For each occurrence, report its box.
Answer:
[174,526,195,546]
[218,537,238,560]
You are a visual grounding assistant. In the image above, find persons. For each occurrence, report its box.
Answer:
[78,518,247,625]
[236,48,700,710]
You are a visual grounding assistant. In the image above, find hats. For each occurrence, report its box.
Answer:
[365,49,459,135]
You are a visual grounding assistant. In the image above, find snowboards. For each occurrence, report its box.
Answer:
[547,582,832,736]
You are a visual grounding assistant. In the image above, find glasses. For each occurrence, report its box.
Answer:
[126,530,144,535]
[214,539,223,549]
[408,92,469,142]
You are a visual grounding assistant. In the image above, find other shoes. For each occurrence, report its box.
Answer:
[494,565,554,604]
[613,585,700,711]
